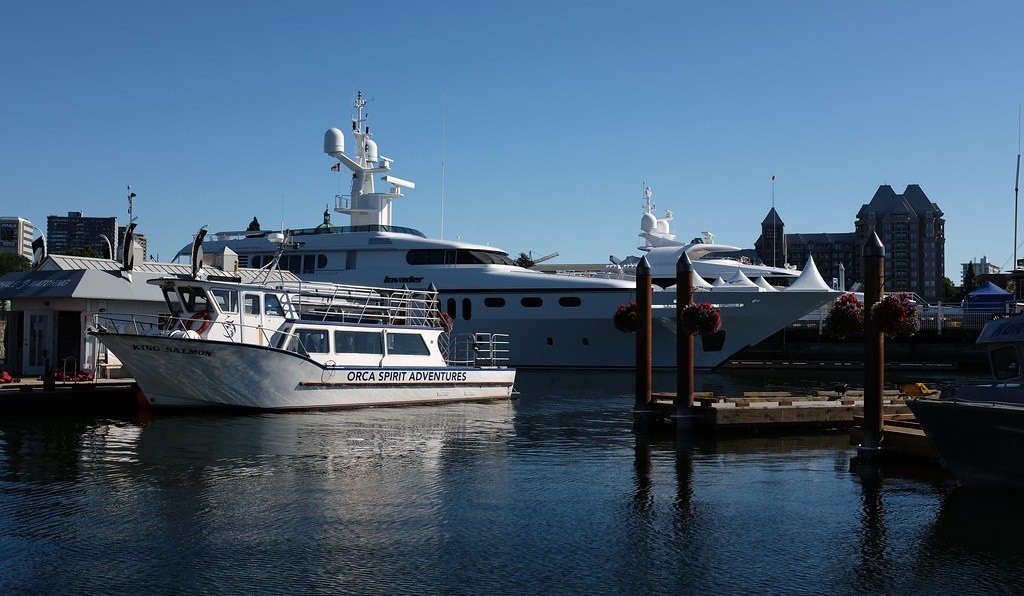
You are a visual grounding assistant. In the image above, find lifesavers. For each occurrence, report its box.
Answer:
[185,312,210,335]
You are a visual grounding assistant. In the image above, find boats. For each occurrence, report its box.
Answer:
[1,91,854,412]
[904,153,1022,482]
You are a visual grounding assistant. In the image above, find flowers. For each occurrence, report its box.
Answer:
[871,292,924,340]
[827,293,863,339]
[612,303,638,334]
[678,302,721,337]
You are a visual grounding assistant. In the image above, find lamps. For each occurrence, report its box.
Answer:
[834,382,848,396]
